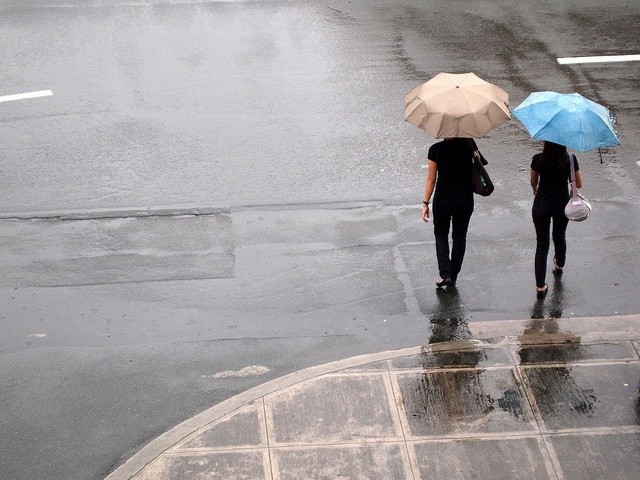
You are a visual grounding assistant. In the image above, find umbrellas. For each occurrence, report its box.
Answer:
[403,71,512,139]
[512,91,622,153]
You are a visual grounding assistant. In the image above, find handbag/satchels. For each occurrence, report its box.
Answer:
[564,154,592,222]
[465,140,494,196]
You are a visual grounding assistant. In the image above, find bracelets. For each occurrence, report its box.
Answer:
[422,200,429,204]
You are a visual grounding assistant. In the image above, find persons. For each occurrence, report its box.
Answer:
[421,138,478,288]
[530,141,582,300]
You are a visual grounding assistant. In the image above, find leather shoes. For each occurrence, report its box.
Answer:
[537,283,548,294]
[436,278,451,286]
